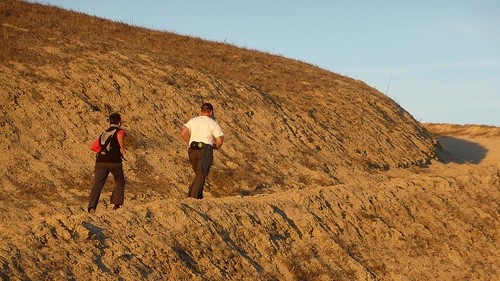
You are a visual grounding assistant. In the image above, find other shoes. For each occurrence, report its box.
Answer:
[88,207,95,212]
[113,205,119,210]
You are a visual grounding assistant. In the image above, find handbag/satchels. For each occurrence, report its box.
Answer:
[190,141,204,150]
